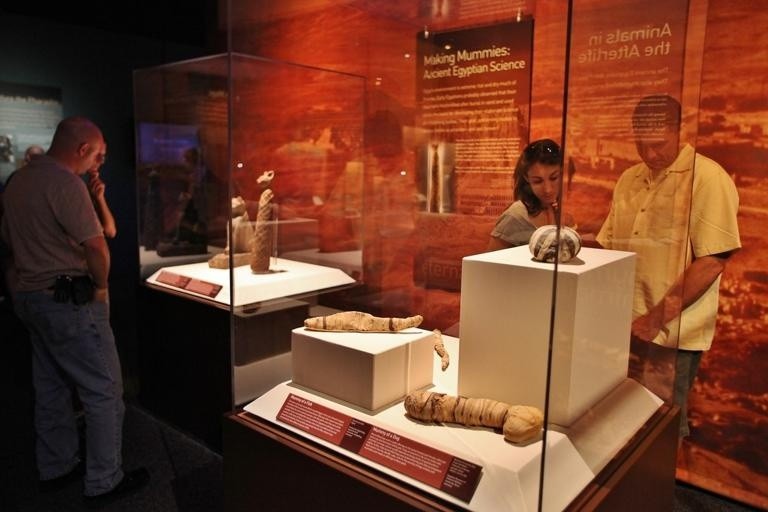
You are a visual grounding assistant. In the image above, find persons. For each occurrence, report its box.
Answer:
[83,138,119,238]
[168,133,230,240]
[273,121,322,184]
[486,138,573,250]
[0,112,150,500]
[22,144,46,165]
[0,135,18,189]
[321,110,416,222]
[592,94,742,442]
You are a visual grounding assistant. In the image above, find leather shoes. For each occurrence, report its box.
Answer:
[86,467,150,499]
